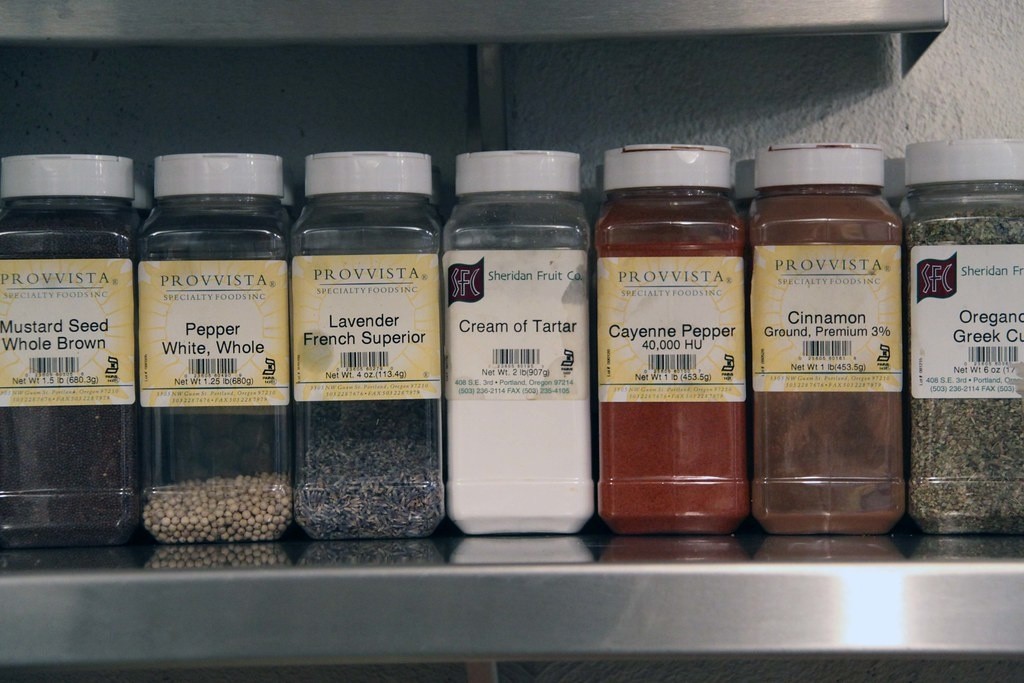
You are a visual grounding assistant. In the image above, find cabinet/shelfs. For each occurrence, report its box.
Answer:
[0,2,1024,683]
